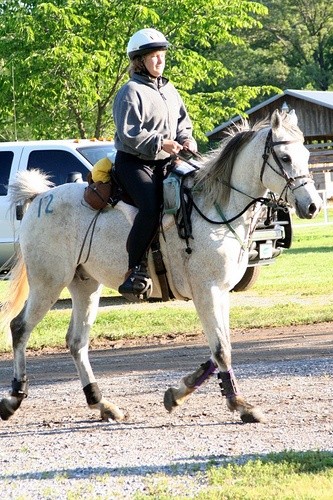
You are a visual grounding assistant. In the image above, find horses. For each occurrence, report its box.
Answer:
[1,108,322,424]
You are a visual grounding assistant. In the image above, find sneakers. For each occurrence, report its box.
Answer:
[118,272,150,293]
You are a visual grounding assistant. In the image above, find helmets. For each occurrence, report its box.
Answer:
[127,28,169,60]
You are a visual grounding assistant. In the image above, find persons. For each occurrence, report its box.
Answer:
[113,28,199,293]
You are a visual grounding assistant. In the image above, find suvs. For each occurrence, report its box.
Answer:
[0,138,288,301]
